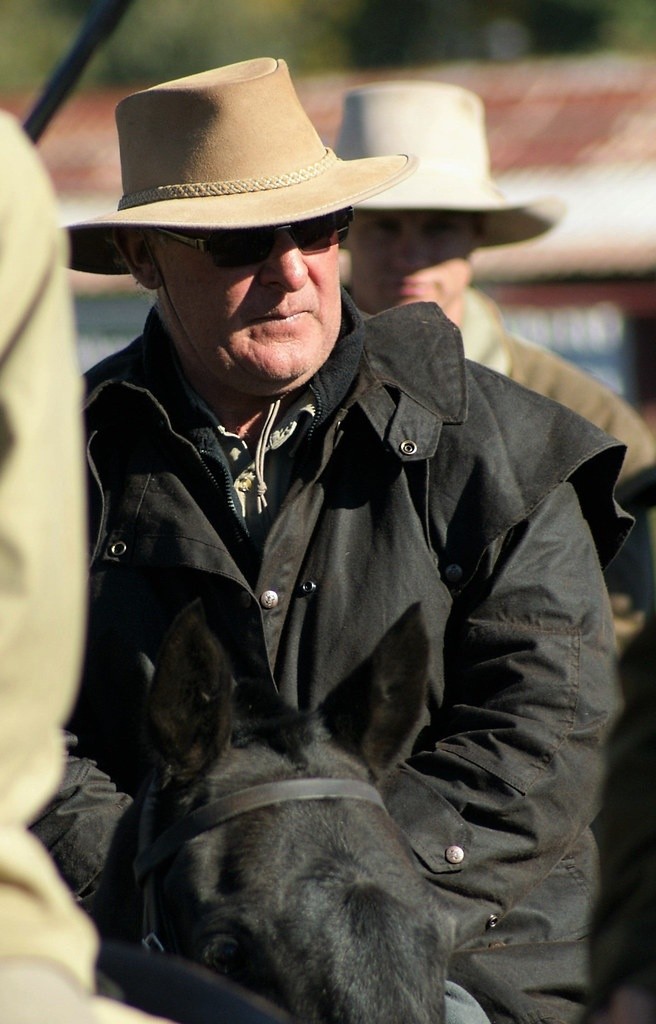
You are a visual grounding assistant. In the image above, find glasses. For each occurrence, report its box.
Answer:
[154,206,354,268]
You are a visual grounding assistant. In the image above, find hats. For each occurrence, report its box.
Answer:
[62,57,420,275]
[334,81,559,244]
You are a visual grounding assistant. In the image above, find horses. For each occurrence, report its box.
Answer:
[82,600,458,1024]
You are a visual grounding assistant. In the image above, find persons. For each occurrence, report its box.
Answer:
[331,80,656,1024]
[29,54,635,1024]
